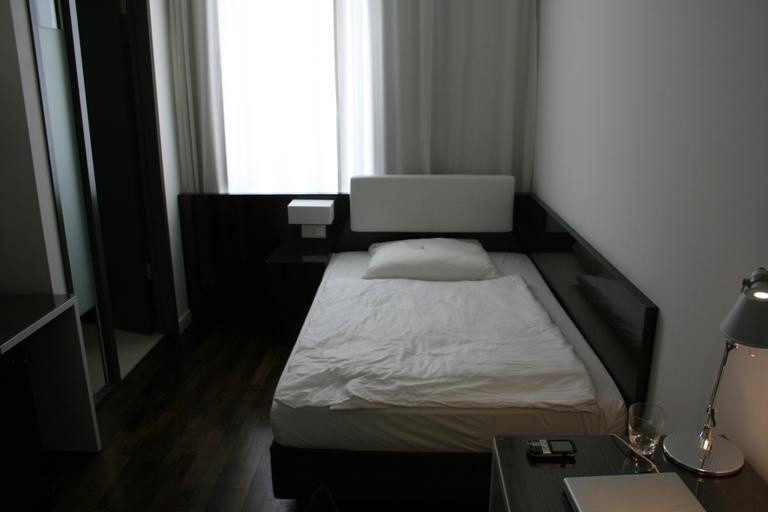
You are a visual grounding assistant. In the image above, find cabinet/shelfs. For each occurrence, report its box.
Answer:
[268,248,327,349]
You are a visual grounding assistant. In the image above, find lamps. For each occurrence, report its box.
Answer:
[660,267,768,476]
[283,197,335,239]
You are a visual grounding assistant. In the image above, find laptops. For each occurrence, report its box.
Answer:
[562,472,708,512]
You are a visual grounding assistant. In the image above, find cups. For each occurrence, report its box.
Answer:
[620,455,654,476]
[627,402,666,455]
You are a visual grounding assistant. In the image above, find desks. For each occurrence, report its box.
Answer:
[487,434,768,511]
[1,293,101,468]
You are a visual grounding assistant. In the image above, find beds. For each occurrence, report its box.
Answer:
[270,173,661,500]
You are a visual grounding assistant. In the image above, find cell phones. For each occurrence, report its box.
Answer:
[528,437,578,458]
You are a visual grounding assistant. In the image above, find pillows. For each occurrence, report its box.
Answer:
[368,237,490,280]
